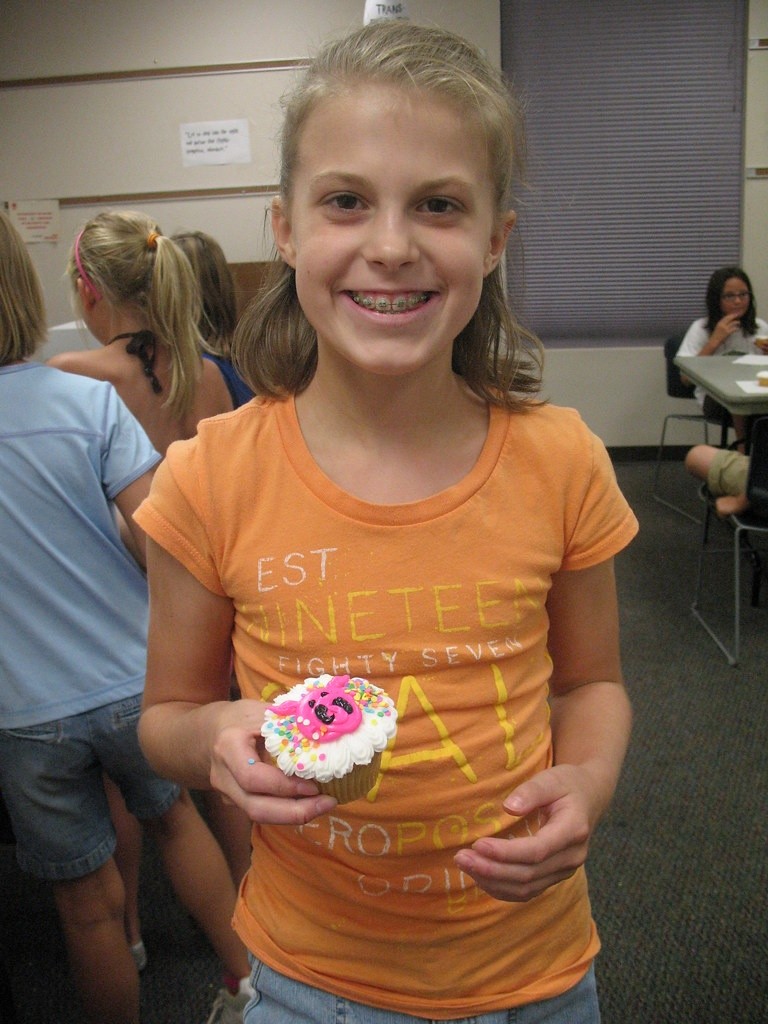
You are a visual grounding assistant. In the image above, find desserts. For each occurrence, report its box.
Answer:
[755,335,768,347]
[757,370,768,387]
[261,673,399,806]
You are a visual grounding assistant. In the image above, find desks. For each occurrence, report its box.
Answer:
[674,355,768,608]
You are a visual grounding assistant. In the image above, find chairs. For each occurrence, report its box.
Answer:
[689,417,768,667]
[649,319,738,527]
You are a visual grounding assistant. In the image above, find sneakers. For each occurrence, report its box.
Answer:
[129,940,147,971]
[206,975,253,1024]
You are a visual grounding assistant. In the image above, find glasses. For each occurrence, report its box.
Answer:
[720,291,754,302]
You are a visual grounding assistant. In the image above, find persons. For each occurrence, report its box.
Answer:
[131,22,647,1024]
[673,266,768,522]
[1,201,256,1024]
